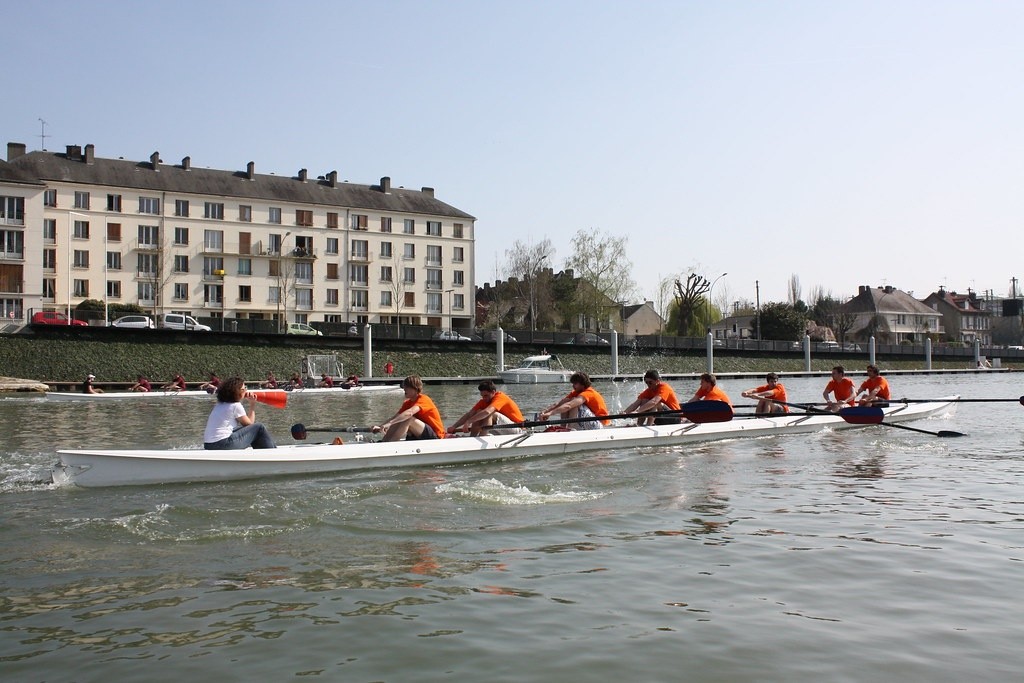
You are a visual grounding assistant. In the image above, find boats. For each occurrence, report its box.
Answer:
[496,353,576,384]
[44,385,402,402]
[55,392,963,489]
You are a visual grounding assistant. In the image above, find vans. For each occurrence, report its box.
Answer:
[1008,346,1024,350]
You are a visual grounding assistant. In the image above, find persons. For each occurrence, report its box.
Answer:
[619,370,686,426]
[204,377,277,450]
[742,372,790,418]
[856,364,891,408]
[539,372,612,431]
[371,374,445,442]
[823,366,856,411]
[200,373,222,391]
[83,374,104,394]
[259,371,277,389]
[318,373,333,388]
[128,374,151,392]
[340,372,358,387]
[291,371,302,388]
[162,372,186,391]
[447,381,527,436]
[688,373,734,414]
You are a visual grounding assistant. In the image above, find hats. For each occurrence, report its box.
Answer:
[87,374,96,378]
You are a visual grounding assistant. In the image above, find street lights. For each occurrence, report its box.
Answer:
[278,231,292,334]
[445,289,455,339]
[710,272,727,333]
[530,256,547,342]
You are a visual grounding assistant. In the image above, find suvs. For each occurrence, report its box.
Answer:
[32,311,88,326]
[158,314,212,331]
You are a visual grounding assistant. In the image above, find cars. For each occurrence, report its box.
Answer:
[107,315,156,329]
[560,333,609,345]
[697,340,729,348]
[783,342,862,352]
[284,323,324,335]
[484,332,517,343]
[433,331,472,341]
[348,326,359,335]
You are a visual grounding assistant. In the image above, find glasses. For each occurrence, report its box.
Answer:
[646,380,653,385]
[244,386,247,390]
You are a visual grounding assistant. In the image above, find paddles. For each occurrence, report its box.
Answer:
[853,396,1024,407]
[129,381,355,391]
[290,423,387,440]
[746,392,966,437]
[623,405,883,425]
[446,399,734,434]
